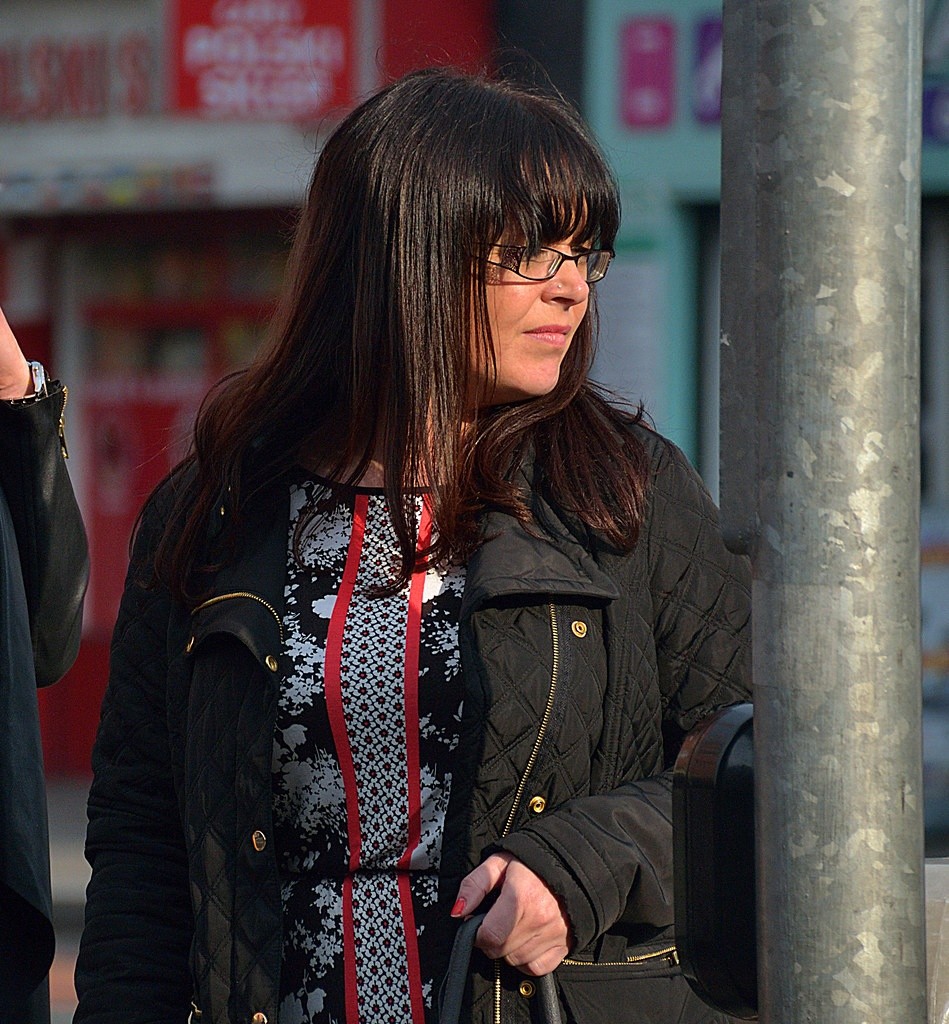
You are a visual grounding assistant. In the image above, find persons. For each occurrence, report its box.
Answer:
[73,68,757,1023]
[1,311,90,1023]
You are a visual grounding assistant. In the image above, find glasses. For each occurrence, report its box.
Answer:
[460,237,615,287]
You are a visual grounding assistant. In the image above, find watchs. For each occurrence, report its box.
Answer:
[0,361,50,405]
